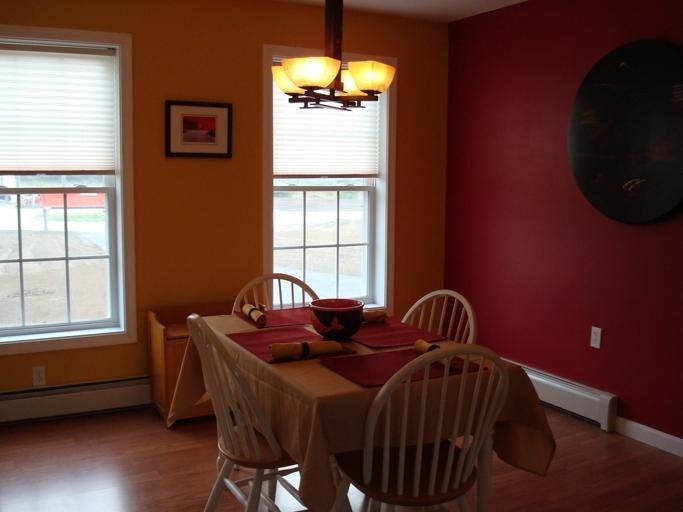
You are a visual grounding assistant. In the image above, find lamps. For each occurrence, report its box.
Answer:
[271,0,396,111]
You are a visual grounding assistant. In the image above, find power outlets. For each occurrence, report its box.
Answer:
[590,325,602,351]
[32,366,47,389]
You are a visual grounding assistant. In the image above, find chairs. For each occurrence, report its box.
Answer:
[232,274,319,314]
[334,344,510,512]
[186,313,310,512]
[401,289,478,344]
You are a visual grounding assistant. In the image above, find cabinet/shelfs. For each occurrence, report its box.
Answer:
[147,300,269,421]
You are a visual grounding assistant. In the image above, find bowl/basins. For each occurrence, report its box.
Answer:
[308,298,363,341]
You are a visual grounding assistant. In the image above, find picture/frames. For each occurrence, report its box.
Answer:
[164,100,233,159]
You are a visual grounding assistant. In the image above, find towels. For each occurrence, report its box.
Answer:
[242,303,267,325]
[267,341,342,360]
[415,339,458,370]
[363,311,386,322]
